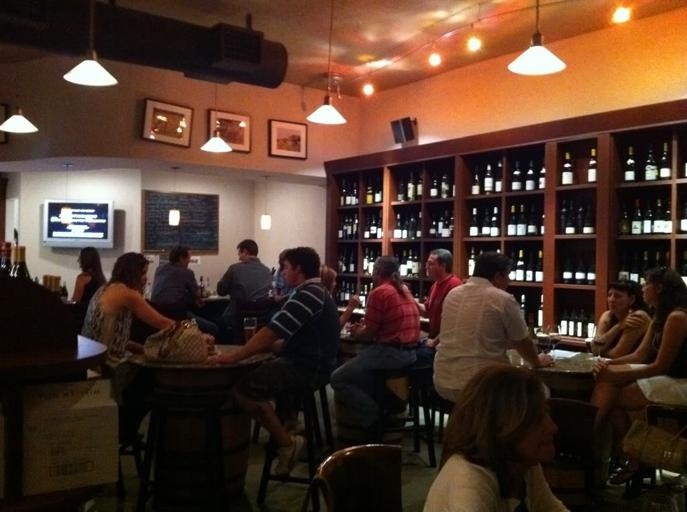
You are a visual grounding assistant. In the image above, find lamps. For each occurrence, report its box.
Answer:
[62,163,72,224]
[391,117,417,143]
[61,0,118,88]
[306,0,347,126]
[0,47,38,134]
[261,175,271,230]
[200,83,232,153]
[169,165,180,227]
[507,0,567,76]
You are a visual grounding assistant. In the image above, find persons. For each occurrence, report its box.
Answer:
[71,238,686,489]
[417,364,574,512]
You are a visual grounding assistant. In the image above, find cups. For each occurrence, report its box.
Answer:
[534,328,551,354]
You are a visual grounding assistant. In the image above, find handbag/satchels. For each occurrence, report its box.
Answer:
[144,319,208,363]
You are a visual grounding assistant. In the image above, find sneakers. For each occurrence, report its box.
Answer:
[610,459,652,484]
[275,435,304,475]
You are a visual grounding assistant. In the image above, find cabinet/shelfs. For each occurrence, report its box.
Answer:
[324,99,687,335]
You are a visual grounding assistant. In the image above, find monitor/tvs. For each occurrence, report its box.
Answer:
[42,199,114,249]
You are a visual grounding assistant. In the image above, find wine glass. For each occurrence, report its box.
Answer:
[545,324,561,361]
[591,324,606,365]
[243,317,258,360]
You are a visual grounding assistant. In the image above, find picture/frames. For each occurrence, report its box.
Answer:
[208,109,253,154]
[140,98,194,149]
[268,119,308,161]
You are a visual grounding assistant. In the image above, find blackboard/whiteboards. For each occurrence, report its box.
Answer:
[141,190,220,255]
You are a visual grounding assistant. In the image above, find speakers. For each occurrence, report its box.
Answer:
[390,116,415,144]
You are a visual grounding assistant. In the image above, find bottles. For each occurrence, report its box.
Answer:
[539,164,546,190]
[198,276,211,297]
[561,151,574,186]
[393,172,422,279]
[625,143,637,184]
[659,142,671,179]
[511,160,523,193]
[526,161,536,192]
[617,195,672,286]
[61,280,67,305]
[507,203,543,330]
[427,173,455,240]
[0,241,31,281]
[587,148,598,183]
[466,163,502,275]
[335,176,382,310]
[559,197,595,340]
[643,142,658,179]
